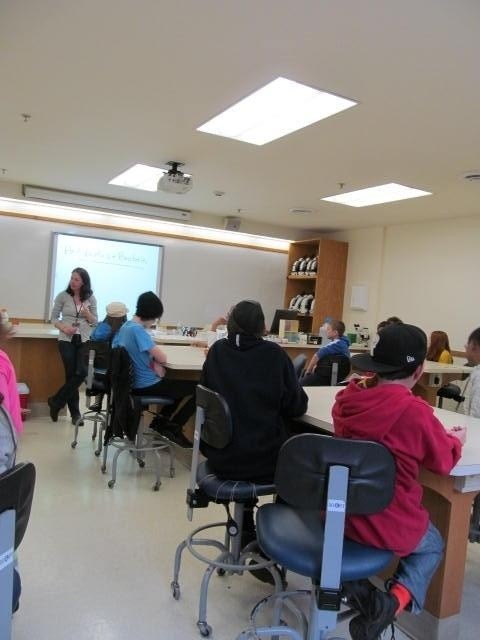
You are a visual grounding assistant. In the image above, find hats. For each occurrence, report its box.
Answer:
[135,292,163,316]
[106,301,129,318]
[350,325,427,373]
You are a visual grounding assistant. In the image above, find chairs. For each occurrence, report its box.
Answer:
[73,337,182,492]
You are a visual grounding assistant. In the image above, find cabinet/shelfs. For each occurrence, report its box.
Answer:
[280,235,349,339]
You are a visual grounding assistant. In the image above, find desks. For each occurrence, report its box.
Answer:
[154,342,211,472]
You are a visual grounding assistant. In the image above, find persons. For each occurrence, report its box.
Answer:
[197,298,309,479]
[110,290,196,450]
[331,316,479,640]
[89,301,131,413]
[0,304,24,437]
[298,319,353,387]
[0,390,23,619]
[47,266,99,427]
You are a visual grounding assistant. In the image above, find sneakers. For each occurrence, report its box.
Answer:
[148,417,167,435]
[48,397,61,422]
[343,578,376,615]
[72,415,84,425]
[349,587,400,640]
[161,423,193,449]
[248,558,287,590]
